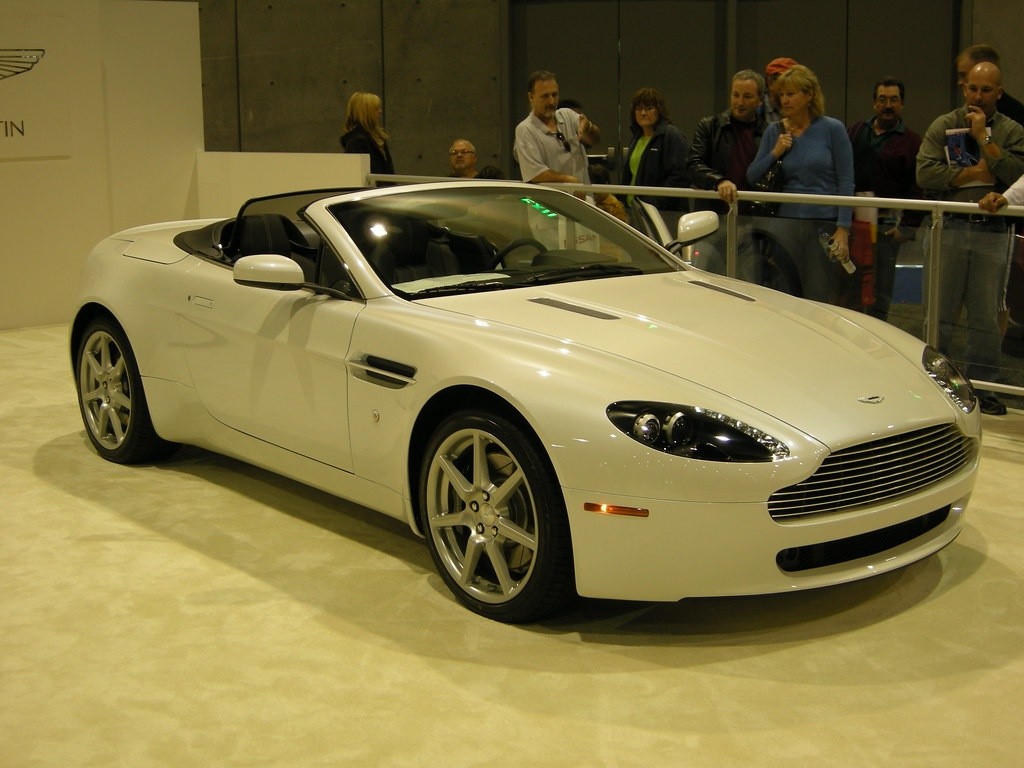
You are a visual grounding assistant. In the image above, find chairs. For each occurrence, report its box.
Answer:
[352,210,461,283]
[218,213,315,284]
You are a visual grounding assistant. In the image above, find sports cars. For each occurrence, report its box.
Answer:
[67,175,986,616]
[656,210,834,303]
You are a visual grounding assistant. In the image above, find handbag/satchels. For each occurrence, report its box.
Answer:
[746,120,784,215]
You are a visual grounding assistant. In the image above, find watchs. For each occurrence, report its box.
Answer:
[977,136,992,147]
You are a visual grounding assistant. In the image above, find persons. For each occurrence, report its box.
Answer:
[836,78,932,322]
[445,138,478,177]
[475,165,506,179]
[956,44,1024,359]
[684,69,768,284]
[620,87,693,239]
[916,61,1024,415]
[587,164,627,262]
[762,56,801,124]
[339,91,397,187]
[746,63,855,306]
[513,70,601,208]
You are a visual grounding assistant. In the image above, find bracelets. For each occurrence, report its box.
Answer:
[583,120,592,137]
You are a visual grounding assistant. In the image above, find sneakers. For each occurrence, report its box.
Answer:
[979,397,1006,414]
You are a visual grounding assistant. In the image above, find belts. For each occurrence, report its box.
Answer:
[944,212,991,223]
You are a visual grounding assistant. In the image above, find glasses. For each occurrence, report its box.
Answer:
[556,132,571,152]
[449,150,474,157]
[876,97,901,103]
